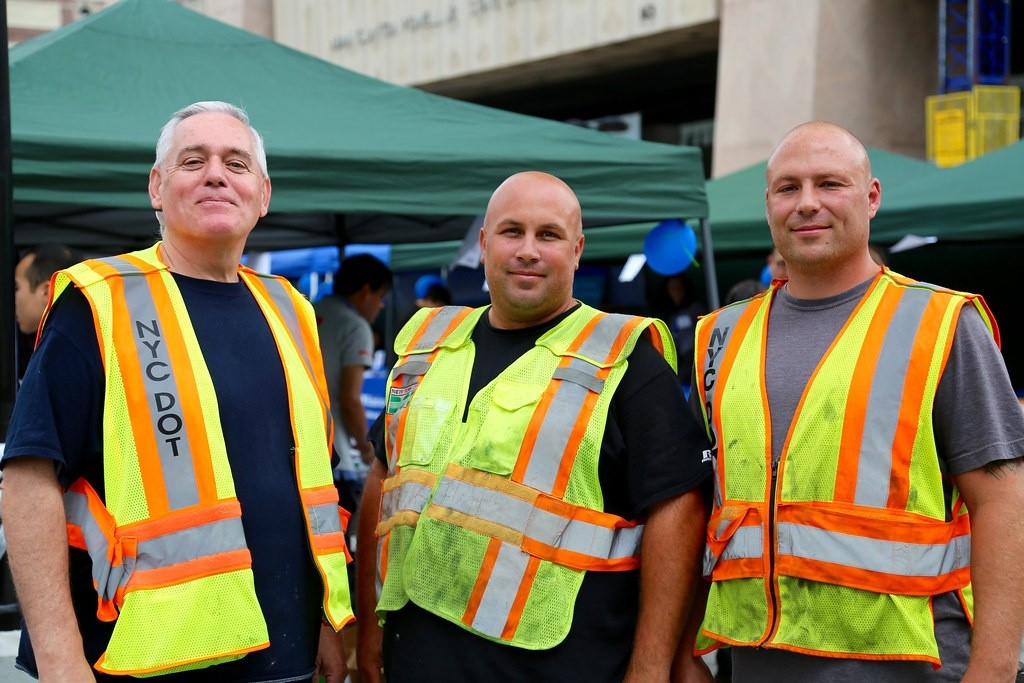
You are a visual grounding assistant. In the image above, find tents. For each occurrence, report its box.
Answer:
[9,0,1023,273]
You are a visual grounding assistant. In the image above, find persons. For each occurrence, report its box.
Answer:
[357,170,715,683]
[669,119,1024,683]
[0,101,356,683]
[313,255,392,468]
[15,245,83,332]
[726,282,767,305]
